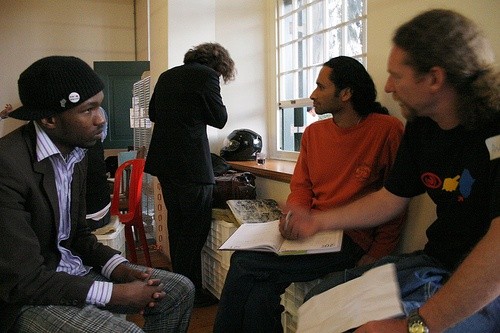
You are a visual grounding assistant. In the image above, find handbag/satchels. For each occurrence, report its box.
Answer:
[211,152,232,177]
[211,171,257,209]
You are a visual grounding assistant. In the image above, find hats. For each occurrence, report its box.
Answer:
[8,56,105,121]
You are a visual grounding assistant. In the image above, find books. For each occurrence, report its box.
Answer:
[217,220,343,256]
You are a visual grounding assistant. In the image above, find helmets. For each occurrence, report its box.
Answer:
[220,129,262,162]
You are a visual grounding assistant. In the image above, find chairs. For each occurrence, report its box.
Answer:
[109,159,152,268]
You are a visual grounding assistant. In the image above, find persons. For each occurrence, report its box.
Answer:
[143,43,235,308]
[211,56,405,333]
[278,9,500,333]
[0,55,196,333]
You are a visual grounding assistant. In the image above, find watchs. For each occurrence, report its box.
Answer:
[407,307,430,333]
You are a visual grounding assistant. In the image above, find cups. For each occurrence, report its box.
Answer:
[255,152,267,169]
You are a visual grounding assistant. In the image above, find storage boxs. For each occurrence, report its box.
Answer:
[202,220,322,333]
[91,215,126,258]
